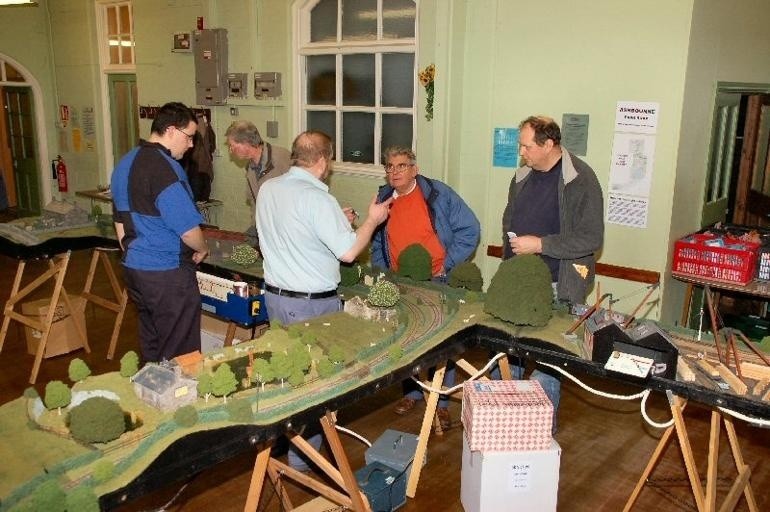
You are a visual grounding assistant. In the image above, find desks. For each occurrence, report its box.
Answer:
[0,202,383,385]
[76,186,222,224]
[671,275,770,328]
[0,288,770,512]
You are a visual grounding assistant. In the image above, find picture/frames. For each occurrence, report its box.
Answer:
[459,380,553,452]
[459,430,562,512]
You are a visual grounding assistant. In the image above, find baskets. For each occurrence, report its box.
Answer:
[673,222,769,288]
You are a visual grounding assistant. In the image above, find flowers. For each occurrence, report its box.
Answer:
[419,64,434,123]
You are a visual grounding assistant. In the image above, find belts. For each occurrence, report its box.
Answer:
[265,284,336,300]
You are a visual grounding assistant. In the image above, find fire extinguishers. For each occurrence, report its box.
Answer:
[50,154,69,193]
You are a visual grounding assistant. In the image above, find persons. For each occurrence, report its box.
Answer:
[255,131,393,474]
[110,103,210,369]
[489,116,604,434]
[225,120,292,237]
[370,144,481,430]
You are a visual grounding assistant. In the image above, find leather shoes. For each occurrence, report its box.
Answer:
[434,407,451,430]
[393,397,414,415]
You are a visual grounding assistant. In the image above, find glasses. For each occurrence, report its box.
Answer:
[383,163,415,173]
[176,127,194,144]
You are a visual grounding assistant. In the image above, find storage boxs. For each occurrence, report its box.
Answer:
[21,294,89,358]
[197,312,270,353]
